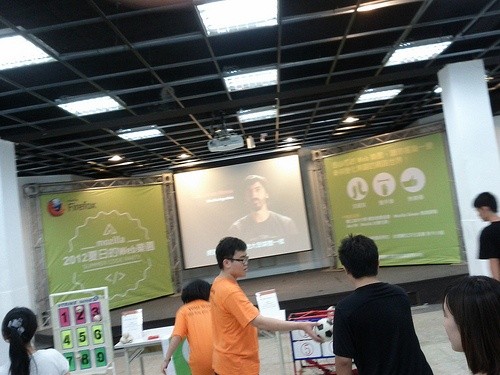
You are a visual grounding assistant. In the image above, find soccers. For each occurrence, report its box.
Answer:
[314,317,333,341]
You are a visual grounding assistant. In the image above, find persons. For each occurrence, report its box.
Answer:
[331,233,435,375]
[159,277,219,375]
[473,192,500,280]
[1,306,72,375]
[441,275,500,375]
[207,236,323,375]
[223,174,302,259]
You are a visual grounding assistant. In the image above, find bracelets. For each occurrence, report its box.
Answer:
[164,357,171,362]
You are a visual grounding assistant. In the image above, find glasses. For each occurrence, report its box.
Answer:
[223,256,249,264]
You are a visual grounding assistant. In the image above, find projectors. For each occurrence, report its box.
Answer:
[207,135,244,152]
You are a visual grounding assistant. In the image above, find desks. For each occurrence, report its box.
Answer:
[113,325,174,375]
